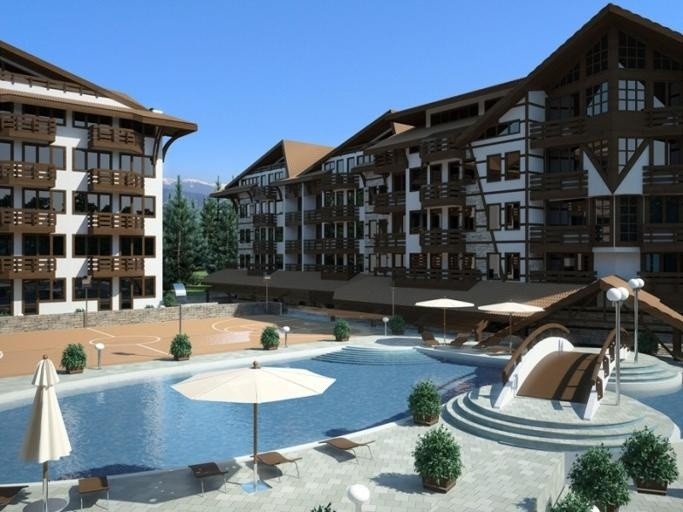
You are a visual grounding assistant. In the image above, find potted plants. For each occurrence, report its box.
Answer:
[409,425,465,494]
[332,316,350,342]
[59,341,86,374]
[169,331,191,361]
[617,425,679,496]
[406,376,441,426]
[566,442,631,512]
[388,313,405,335]
[259,325,281,350]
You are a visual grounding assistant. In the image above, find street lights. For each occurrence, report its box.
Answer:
[94,342,105,370]
[344,483,372,512]
[81,278,93,326]
[381,316,389,338]
[281,326,291,348]
[605,285,630,407]
[264,276,271,313]
[628,276,645,364]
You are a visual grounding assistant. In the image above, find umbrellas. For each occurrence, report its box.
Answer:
[20,354,71,512]
[414,295,475,346]
[476,300,544,355]
[171,361,336,492]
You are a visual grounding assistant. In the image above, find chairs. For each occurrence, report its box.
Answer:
[77,474,110,512]
[188,460,229,498]
[0,484,29,512]
[419,328,503,350]
[318,437,375,465]
[250,452,303,481]
